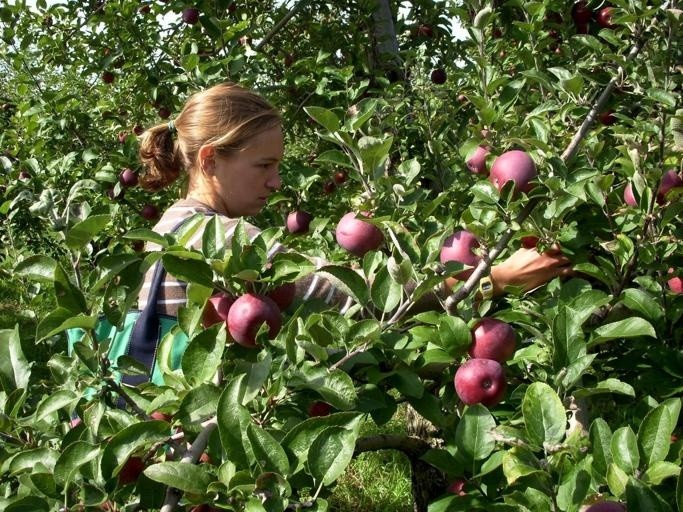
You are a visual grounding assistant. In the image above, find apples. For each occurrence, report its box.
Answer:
[0,1,683,512]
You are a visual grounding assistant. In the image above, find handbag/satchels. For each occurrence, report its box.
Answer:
[59,309,210,460]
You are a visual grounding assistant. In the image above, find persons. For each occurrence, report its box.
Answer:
[58,82,577,473]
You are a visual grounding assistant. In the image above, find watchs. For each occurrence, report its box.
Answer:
[479,272,493,303]
